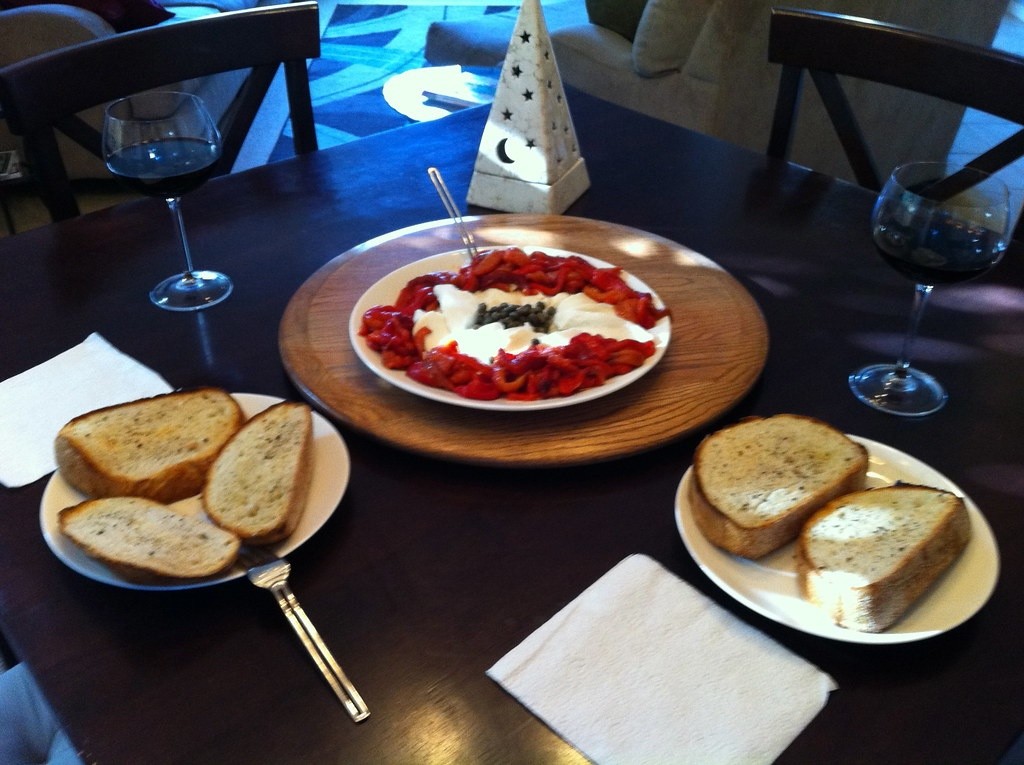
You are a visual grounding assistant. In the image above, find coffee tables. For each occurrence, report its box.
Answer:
[383,63,504,123]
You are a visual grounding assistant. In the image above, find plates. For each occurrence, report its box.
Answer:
[382,65,502,122]
[38,392,350,591]
[349,245,672,410]
[673,433,1000,643]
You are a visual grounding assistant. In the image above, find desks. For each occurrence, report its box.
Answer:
[0,82,1024,765]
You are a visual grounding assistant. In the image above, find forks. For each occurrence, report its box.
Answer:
[238,540,371,724]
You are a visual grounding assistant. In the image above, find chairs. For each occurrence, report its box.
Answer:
[1,0,321,236]
[765,0,1024,244]
[0,0,321,218]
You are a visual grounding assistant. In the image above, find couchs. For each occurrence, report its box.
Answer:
[424,0,1011,186]
[0,0,334,192]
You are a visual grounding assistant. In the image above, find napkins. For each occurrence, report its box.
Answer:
[0,332,174,487]
[486,554,839,765]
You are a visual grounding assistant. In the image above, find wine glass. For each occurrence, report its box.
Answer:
[848,161,1012,418]
[102,89,235,310]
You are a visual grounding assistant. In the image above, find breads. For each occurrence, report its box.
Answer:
[794,480,970,633]
[201,398,313,546]
[57,493,243,587]
[687,412,869,558]
[53,384,248,503]
